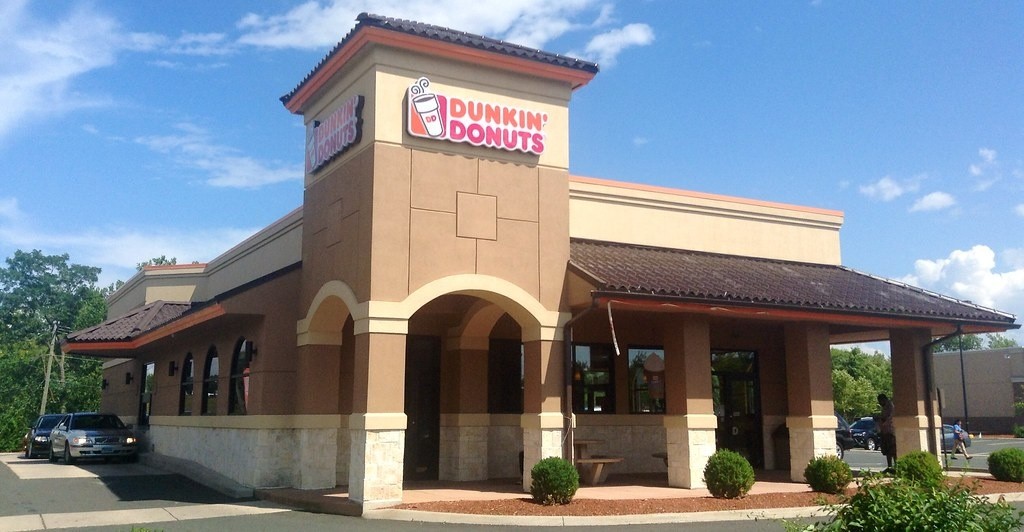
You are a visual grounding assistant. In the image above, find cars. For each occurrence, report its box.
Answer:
[49,412,137,464]
[26,413,65,459]
[941,423,971,453]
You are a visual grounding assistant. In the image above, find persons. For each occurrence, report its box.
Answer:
[872,394,897,475]
[951,419,973,460]
[515,451,524,485]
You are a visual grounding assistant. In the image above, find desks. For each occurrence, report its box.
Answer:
[574,438,606,486]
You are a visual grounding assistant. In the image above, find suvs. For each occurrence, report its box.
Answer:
[834,410,853,461]
[849,416,882,450]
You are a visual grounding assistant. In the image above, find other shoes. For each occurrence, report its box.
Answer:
[966,456,973,459]
[951,456,958,459]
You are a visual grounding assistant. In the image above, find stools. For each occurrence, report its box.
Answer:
[576,456,624,485]
